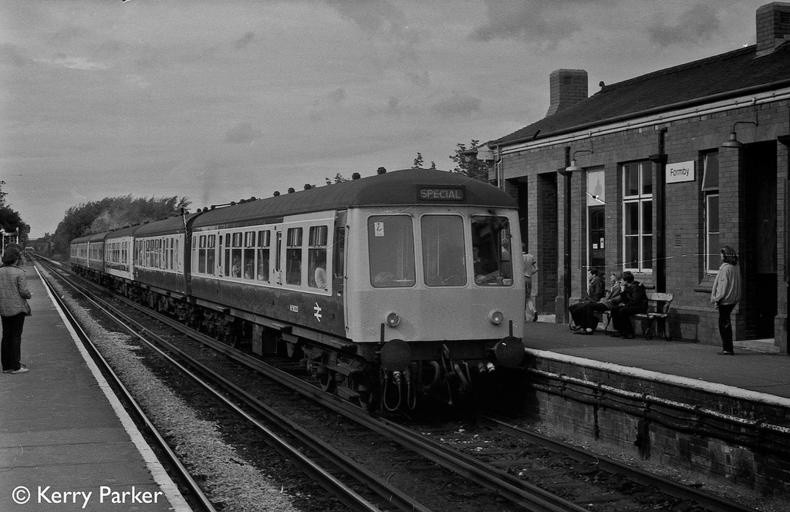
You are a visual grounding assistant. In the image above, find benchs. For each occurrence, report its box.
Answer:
[569,292,673,342]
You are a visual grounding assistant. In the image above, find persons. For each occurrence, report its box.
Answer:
[472,243,499,285]
[522,241,539,323]
[499,239,513,280]
[0,247,33,373]
[0,243,22,267]
[572,270,625,334]
[708,245,743,356]
[568,267,604,334]
[609,271,649,339]
[228,255,327,290]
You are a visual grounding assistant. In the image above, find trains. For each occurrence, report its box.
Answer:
[67,165,531,421]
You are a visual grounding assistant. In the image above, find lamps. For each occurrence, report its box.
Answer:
[565,148,593,172]
[719,121,759,148]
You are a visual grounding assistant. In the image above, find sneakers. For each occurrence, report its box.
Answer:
[717,350,735,355]
[532,311,538,322]
[2,368,29,374]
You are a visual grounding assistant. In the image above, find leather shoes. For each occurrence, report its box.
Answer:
[572,328,593,335]
